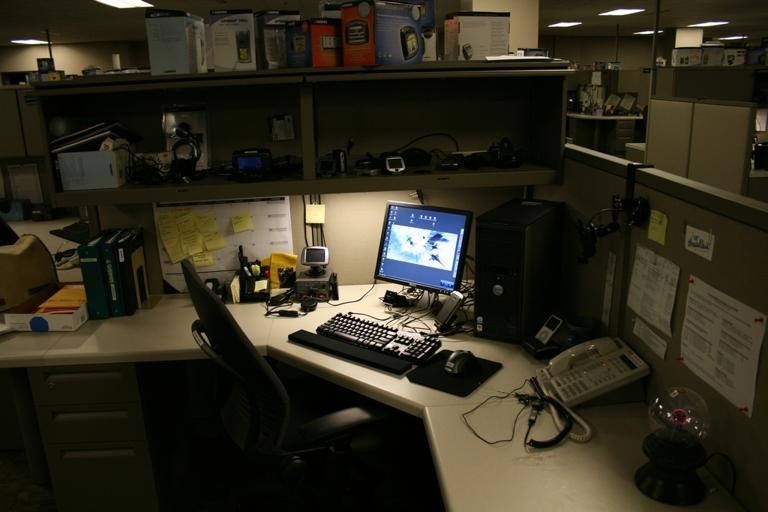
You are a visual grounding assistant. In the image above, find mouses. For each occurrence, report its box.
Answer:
[444,350,472,375]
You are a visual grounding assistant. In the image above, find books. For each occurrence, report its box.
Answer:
[52,121,140,149]
[50,129,122,154]
[47,119,109,147]
[35,280,87,314]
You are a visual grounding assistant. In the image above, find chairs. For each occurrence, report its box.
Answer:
[181,259,372,512]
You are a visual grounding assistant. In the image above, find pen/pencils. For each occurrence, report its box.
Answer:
[239,245,261,277]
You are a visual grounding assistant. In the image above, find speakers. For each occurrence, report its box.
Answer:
[436,290,465,332]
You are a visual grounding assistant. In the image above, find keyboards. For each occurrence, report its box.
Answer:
[317,312,443,368]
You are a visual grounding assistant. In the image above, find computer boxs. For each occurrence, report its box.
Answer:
[474,198,566,347]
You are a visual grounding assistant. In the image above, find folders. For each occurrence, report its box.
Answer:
[77,227,168,320]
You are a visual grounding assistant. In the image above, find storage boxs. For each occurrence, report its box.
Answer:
[56,149,133,191]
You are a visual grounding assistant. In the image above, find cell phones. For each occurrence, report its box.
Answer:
[440,153,462,170]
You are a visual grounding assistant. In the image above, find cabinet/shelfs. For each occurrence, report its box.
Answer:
[0,0,768,512]
[748,169,768,202]
[26,351,163,511]
[604,120,635,149]
[18,69,577,207]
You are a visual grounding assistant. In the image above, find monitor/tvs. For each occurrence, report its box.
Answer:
[375,199,472,312]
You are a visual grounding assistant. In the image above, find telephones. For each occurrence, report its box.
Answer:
[542,335,650,408]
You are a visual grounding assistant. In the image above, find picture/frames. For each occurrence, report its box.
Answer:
[0,155,56,207]
[160,103,213,170]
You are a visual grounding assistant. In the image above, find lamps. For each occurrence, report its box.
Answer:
[700,36,725,46]
[675,28,703,48]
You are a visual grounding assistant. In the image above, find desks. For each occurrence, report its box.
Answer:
[1,279,747,510]
[567,112,644,152]
